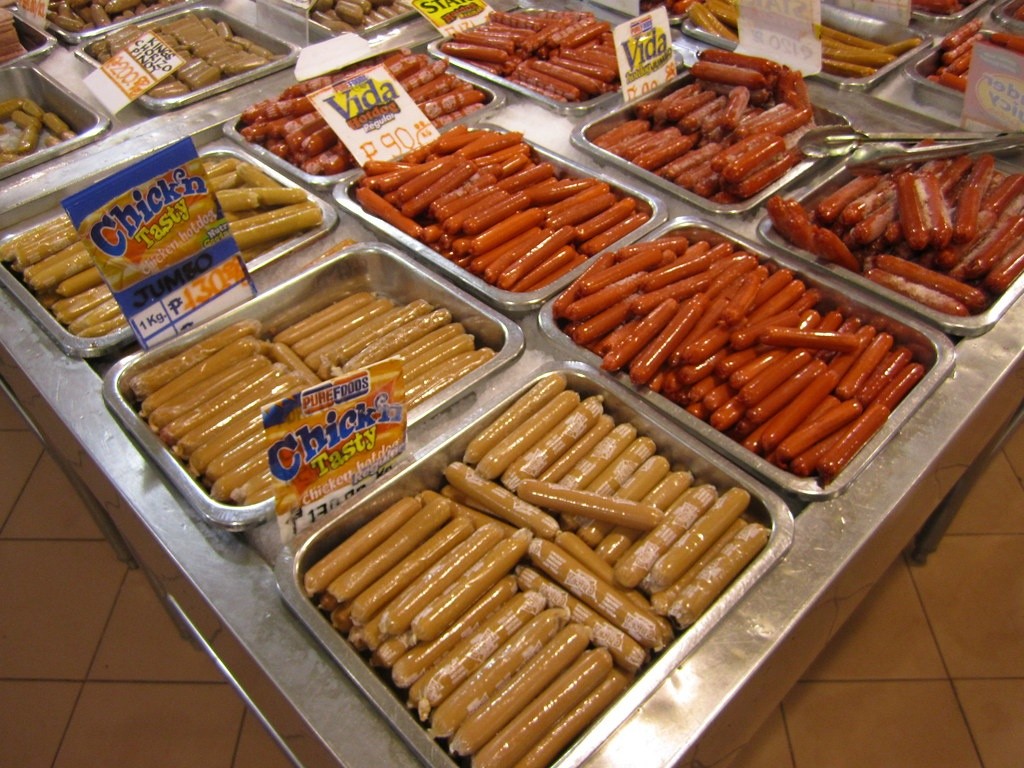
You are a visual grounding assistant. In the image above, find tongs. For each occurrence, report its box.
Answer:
[798,124,1024,175]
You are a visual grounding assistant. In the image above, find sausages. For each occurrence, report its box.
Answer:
[1,0,1024,767]
